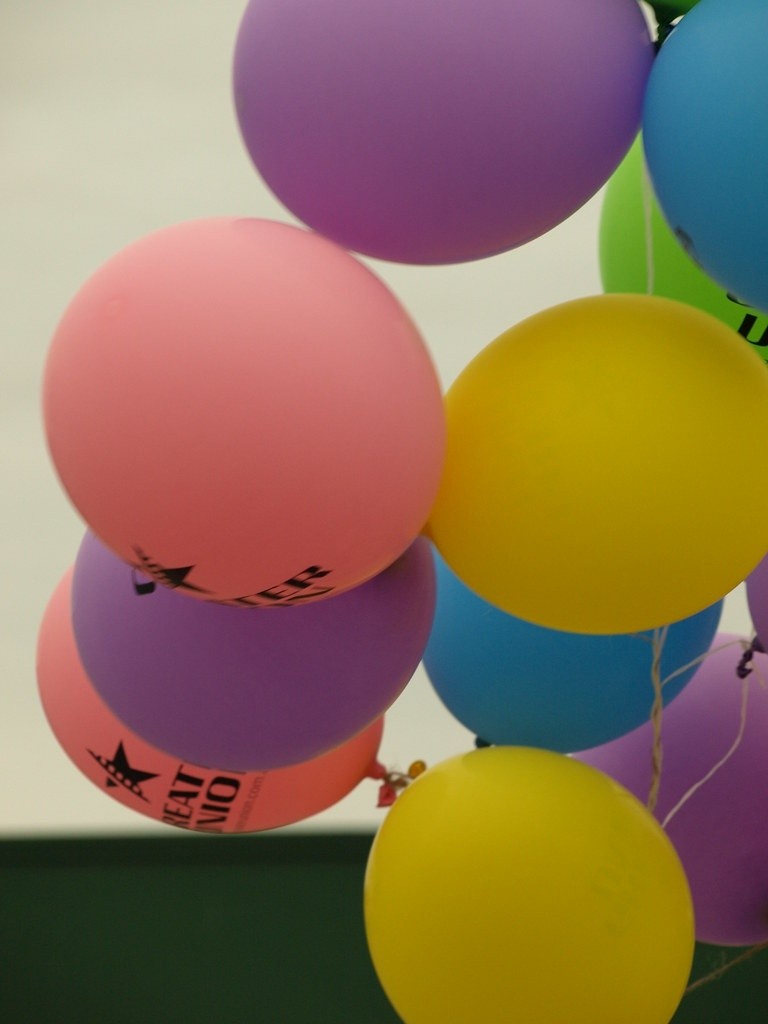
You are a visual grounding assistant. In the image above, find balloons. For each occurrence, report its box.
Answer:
[32,0,768,1024]
